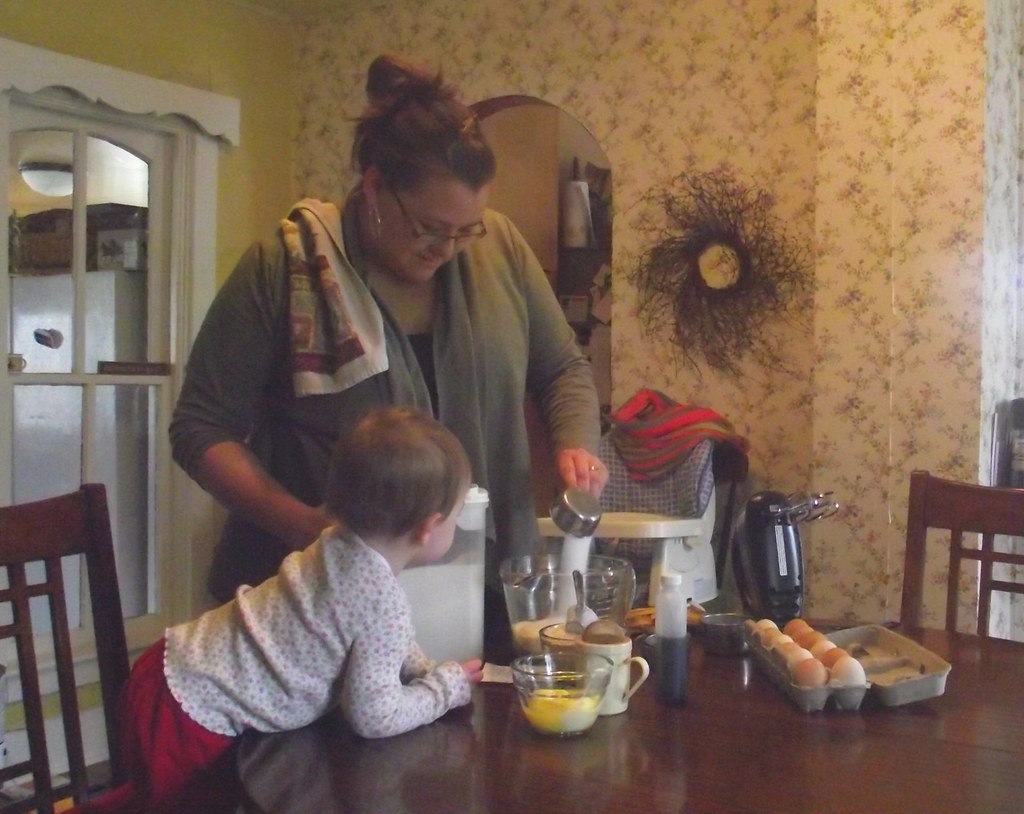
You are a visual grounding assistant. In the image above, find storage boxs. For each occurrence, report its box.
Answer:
[13,225,148,273]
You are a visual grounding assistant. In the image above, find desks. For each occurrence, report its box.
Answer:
[220,616,1024,814]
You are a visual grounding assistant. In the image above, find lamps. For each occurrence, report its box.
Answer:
[19,160,74,196]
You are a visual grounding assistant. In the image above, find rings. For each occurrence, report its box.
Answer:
[591,464,598,471]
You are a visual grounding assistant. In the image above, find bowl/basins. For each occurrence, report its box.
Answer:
[498,553,637,660]
[510,653,615,738]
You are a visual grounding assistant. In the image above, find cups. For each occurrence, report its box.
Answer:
[550,487,603,538]
[574,636,650,716]
[8,354,26,372]
[539,624,584,690]
[699,612,754,656]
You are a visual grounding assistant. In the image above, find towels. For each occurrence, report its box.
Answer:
[278,196,391,398]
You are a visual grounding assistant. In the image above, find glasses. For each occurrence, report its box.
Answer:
[376,166,487,245]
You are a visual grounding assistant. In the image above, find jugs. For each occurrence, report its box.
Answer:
[398,483,490,666]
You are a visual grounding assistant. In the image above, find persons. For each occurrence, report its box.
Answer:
[168,54,609,666]
[56,406,484,814]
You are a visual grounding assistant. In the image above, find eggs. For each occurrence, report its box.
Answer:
[754,618,866,687]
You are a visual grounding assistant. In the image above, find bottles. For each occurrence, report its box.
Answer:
[654,573,688,639]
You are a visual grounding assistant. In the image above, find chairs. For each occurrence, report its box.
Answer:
[898,470,1024,642]
[0,484,131,814]
[530,427,718,609]
